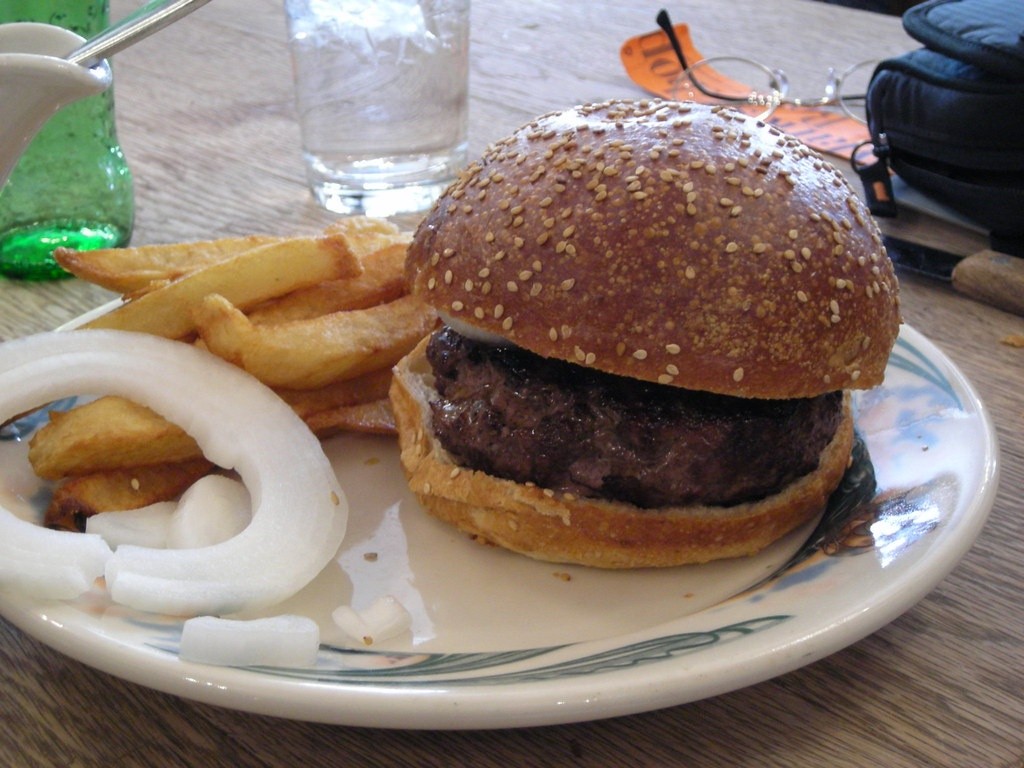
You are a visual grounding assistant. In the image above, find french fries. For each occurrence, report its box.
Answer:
[25,215,436,534]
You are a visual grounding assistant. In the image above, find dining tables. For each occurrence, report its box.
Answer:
[0,0,1023,767]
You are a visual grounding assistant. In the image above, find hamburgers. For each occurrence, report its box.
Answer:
[384,100,902,567]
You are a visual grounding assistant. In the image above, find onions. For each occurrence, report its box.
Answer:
[0,329,414,668]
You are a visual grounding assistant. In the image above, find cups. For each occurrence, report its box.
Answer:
[283,0,470,217]
[0,0,136,281]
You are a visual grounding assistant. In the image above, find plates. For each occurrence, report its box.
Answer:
[1,232,997,731]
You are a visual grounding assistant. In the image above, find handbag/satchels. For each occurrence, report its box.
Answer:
[850,0,1024,258]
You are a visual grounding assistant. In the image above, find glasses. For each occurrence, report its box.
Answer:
[655,8,893,126]
